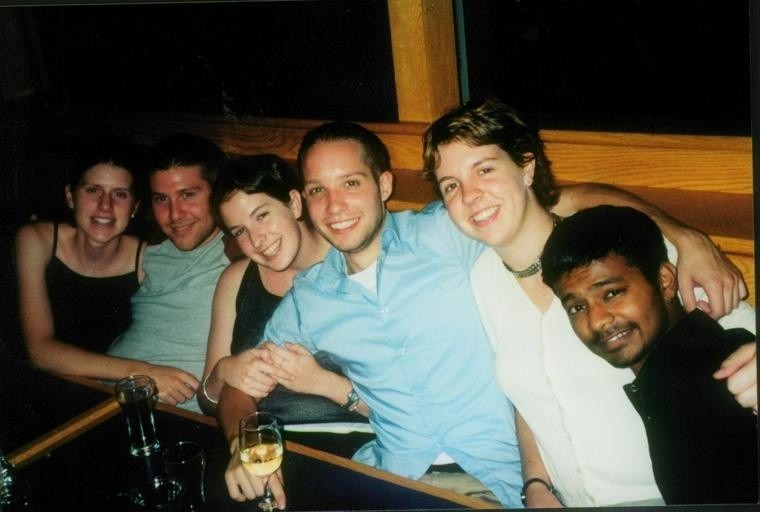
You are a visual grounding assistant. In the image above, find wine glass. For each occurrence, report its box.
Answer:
[236,411,285,512]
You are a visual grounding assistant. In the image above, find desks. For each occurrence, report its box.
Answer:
[0,358,487,511]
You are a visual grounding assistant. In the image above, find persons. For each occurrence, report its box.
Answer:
[15,94,757,512]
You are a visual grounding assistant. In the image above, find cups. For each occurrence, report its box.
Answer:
[116,376,161,458]
[155,442,208,512]
